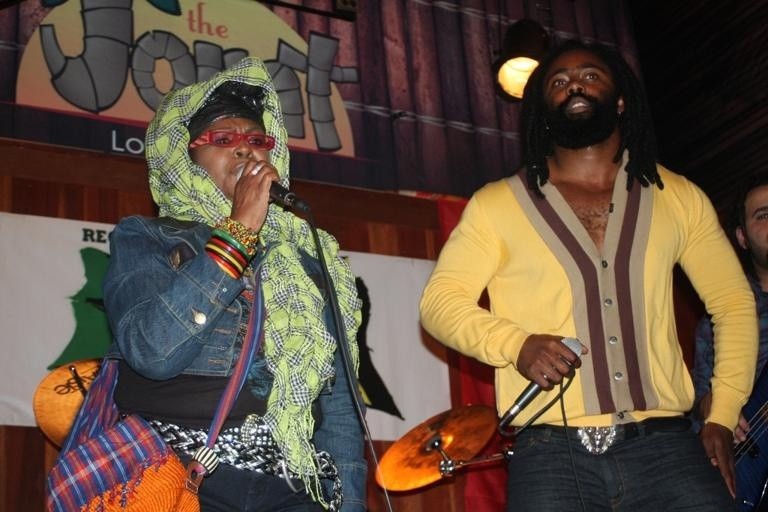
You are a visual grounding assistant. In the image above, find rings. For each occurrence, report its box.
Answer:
[251,166,262,175]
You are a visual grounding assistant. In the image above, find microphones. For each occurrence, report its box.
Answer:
[236,168,310,214]
[498,336,584,441]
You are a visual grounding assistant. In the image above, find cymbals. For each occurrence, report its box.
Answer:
[375,405,498,492]
[33,358,104,450]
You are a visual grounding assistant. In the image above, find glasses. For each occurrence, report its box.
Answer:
[184,130,278,153]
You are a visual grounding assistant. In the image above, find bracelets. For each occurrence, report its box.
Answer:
[205,216,257,280]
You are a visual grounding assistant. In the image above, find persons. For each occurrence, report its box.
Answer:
[420,38,760,510]
[101,55,374,512]
[697,177,767,509]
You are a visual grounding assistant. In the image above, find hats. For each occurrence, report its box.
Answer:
[174,84,268,138]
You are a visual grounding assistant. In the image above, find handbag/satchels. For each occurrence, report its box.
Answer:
[43,413,201,512]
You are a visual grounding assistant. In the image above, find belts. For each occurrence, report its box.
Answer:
[518,414,694,457]
